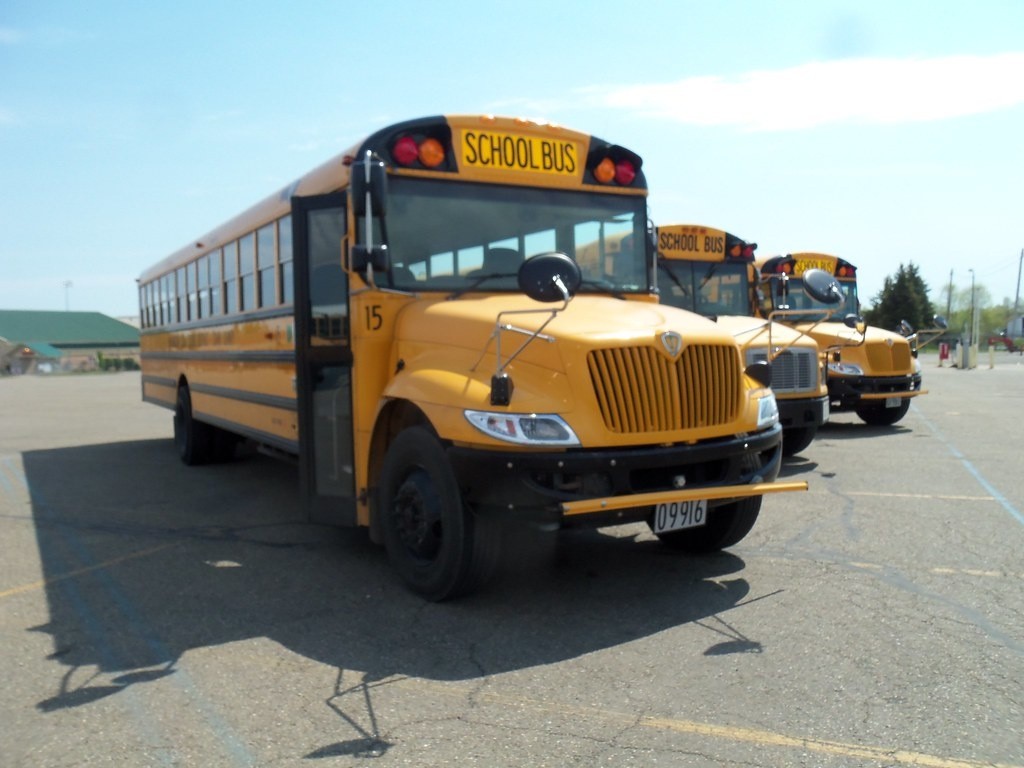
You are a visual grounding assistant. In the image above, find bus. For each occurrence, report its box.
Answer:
[134,114,810,604]
[415,224,867,458]
[669,253,948,427]
[894,320,922,389]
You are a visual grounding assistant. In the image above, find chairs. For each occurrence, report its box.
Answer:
[388,248,523,288]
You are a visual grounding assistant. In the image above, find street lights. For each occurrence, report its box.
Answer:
[968,268,975,346]
